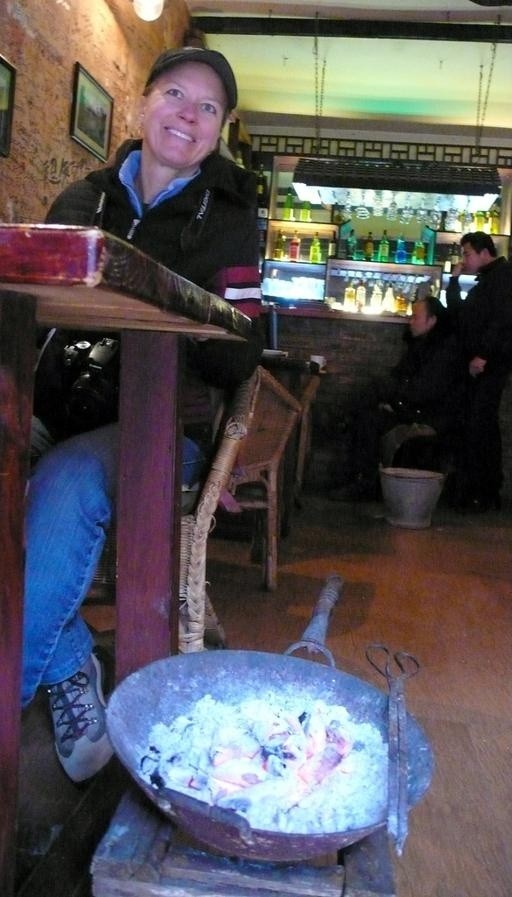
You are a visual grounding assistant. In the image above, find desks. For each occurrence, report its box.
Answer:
[1,222,252,897]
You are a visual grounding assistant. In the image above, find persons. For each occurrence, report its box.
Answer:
[0,43,262,782]
[445,231,511,512]
[321,292,478,503]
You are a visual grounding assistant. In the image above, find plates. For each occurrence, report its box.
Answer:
[262,349,286,356]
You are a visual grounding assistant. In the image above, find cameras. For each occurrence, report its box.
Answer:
[62,337,120,428]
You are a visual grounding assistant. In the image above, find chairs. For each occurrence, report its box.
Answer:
[232,363,323,589]
[79,350,260,668]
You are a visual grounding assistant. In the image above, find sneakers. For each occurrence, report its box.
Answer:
[46,644,113,786]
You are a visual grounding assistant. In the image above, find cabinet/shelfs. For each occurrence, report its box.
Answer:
[247,150,512,323]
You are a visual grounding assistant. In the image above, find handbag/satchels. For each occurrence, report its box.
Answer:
[59,338,210,427]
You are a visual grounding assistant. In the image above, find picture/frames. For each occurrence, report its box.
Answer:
[68,62,114,165]
[0,53,17,154]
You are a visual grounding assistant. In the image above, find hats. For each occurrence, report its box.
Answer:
[146,46,237,111]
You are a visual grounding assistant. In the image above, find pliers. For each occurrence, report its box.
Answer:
[364,643,421,857]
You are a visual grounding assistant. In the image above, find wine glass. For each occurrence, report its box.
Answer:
[341,190,472,231]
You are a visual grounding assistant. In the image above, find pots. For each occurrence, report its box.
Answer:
[104,575,435,862]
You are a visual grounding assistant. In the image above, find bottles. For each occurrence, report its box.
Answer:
[255,163,268,208]
[259,230,265,257]
[272,229,426,265]
[300,201,311,221]
[235,151,245,169]
[442,241,459,274]
[344,279,395,311]
[473,203,501,235]
[282,187,294,220]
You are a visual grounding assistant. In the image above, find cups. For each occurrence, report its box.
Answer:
[397,295,409,316]
[310,355,327,371]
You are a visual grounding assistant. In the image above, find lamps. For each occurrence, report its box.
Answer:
[293,11,501,215]
